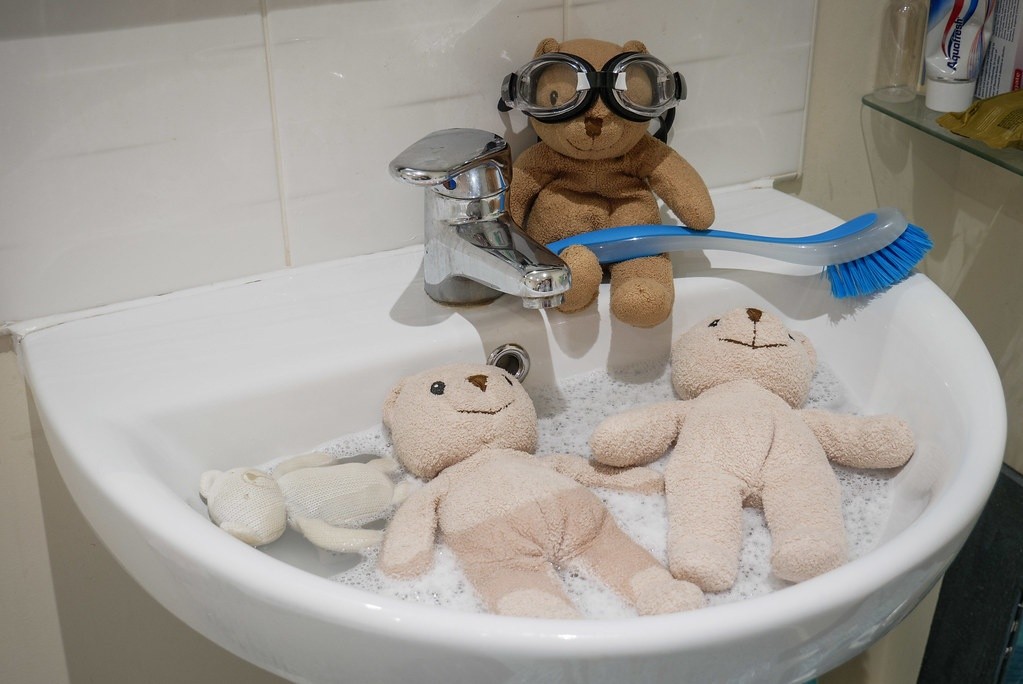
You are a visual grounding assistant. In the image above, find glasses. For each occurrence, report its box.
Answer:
[497,51,687,124]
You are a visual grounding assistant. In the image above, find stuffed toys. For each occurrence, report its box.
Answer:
[379,365,705,625]
[592,306,915,591]
[202,456,414,553]
[509,37,716,328]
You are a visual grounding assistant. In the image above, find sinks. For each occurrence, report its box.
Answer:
[6,184,1011,684]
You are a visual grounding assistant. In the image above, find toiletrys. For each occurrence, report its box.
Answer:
[922,0,998,115]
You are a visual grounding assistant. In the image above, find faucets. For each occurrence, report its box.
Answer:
[386,123,572,312]
